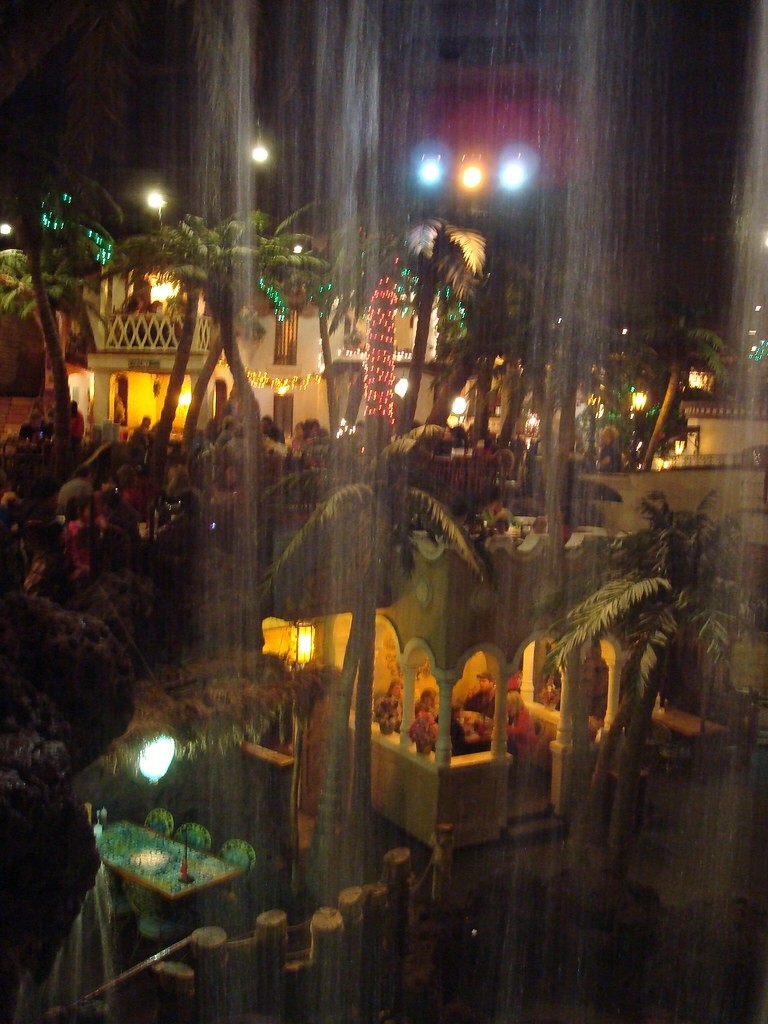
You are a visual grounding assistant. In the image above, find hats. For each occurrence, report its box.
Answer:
[477,672,495,680]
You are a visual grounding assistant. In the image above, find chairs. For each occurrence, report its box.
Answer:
[215,835,259,908]
[141,807,174,835]
[170,822,213,853]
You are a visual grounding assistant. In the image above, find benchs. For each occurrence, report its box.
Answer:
[494,705,573,814]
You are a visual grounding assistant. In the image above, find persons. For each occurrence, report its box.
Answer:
[433,696,468,757]
[488,688,536,787]
[0,487,144,1024]
[382,678,404,727]
[0,381,637,632]
[466,671,504,717]
[414,685,437,744]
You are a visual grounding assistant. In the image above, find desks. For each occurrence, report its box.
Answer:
[90,818,245,938]
[649,701,728,742]
